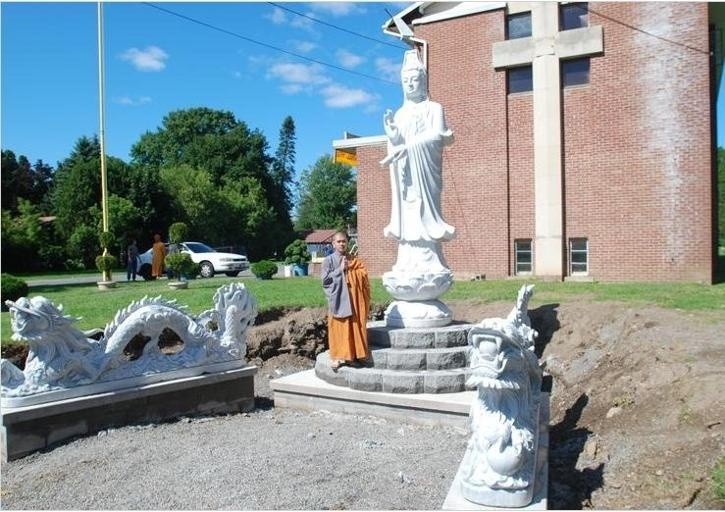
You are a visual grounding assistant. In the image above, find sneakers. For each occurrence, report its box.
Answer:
[331,359,362,369]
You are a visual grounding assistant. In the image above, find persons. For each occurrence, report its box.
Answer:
[127,238,142,281]
[377,49,456,273]
[151,234,167,280]
[321,230,370,369]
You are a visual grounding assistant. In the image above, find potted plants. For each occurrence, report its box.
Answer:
[94,232,119,290]
[163,252,200,290]
[282,239,311,278]
[250,259,279,281]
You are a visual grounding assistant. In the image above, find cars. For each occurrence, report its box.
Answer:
[137,242,249,281]
